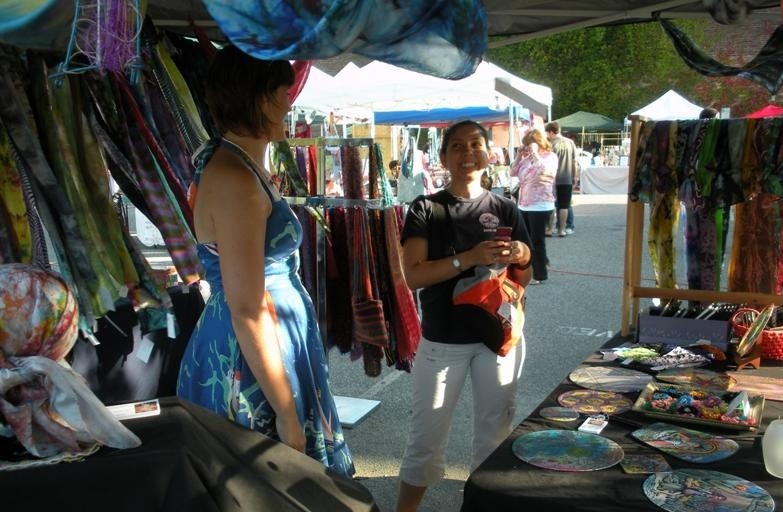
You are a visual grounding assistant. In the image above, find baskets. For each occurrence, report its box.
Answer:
[728,307,783,364]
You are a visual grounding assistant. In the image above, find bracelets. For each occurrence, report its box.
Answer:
[516,254,532,271]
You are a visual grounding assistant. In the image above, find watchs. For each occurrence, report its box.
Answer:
[450,255,465,272]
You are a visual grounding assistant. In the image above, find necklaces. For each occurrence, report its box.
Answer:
[220,137,275,185]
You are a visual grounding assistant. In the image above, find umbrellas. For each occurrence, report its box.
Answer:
[549,111,618,148]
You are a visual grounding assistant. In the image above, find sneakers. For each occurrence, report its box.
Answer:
[566,228,574,235]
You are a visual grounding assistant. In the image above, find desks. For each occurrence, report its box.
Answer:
[461,329,782,511]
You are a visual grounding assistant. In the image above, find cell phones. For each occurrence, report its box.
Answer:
[497,227,512,249]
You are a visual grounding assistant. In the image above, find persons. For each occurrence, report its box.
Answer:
[389,160,402,197]
[510,131,559,285]
[176,46,357,480]
[700,107,721,121]
[486,143,511,166]
[395,120,534,512]
[545,122,615,236]
